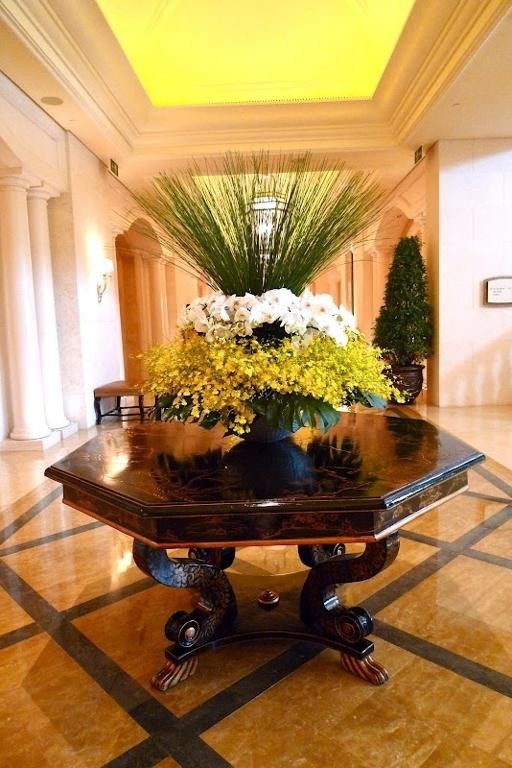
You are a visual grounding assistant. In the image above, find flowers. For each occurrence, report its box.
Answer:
[125,291,413,441]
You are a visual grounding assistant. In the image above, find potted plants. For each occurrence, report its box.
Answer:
[371,237,432,406]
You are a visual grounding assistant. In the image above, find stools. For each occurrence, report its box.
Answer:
[94,381,162,426]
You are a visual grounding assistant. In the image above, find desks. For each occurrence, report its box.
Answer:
[43,411,486,692]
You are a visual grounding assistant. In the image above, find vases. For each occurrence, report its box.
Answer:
[222,410,310,440]
